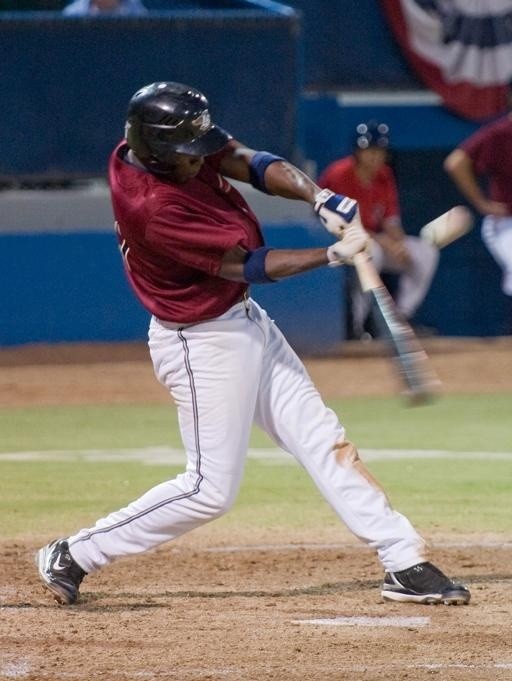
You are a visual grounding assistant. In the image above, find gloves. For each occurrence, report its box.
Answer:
[325,225,371,269]
[312,185,364,239]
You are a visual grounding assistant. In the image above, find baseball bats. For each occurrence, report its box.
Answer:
[344,226,437,406]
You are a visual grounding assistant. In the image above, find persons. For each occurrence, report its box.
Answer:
[315,118,441,341]
[35,79,473,607]
[61,0,152,17]
[442,106,511,297]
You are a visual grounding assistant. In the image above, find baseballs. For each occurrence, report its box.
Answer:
[422,205,472,248]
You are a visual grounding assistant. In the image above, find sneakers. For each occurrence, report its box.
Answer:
[380,561,473,607]
[33,536,87,607]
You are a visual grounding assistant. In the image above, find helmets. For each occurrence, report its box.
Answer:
[123,80,234,161]
[353,117,390,153]
